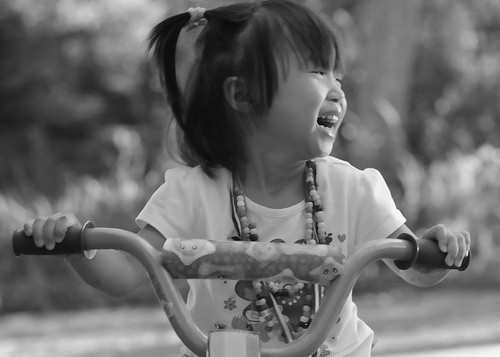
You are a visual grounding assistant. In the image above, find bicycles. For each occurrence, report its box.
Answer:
[11,219,470,354]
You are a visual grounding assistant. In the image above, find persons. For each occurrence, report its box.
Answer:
[23,1,469,357]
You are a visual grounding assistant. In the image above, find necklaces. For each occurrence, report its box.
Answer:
[229,158,327,357]
